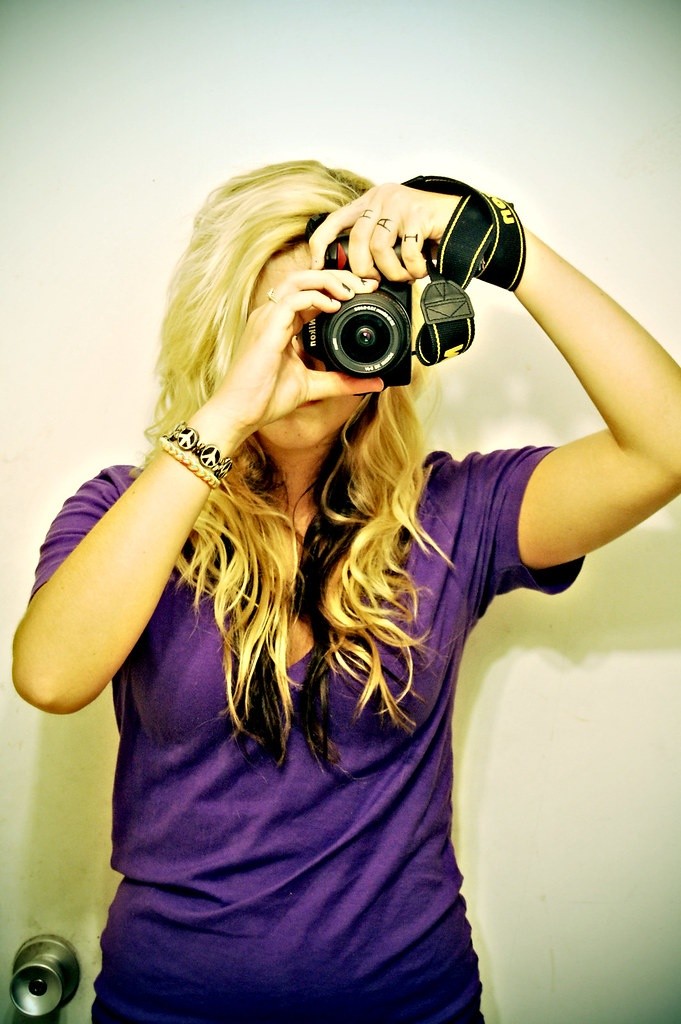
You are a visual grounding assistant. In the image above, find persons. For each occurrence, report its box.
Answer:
[12,161,681,1024]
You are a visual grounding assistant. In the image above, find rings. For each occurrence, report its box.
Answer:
[265,288,283,304]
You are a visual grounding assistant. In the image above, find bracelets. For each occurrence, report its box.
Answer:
[157,421,234,490]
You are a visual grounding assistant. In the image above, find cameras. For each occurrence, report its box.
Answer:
[295,212,413,388]
